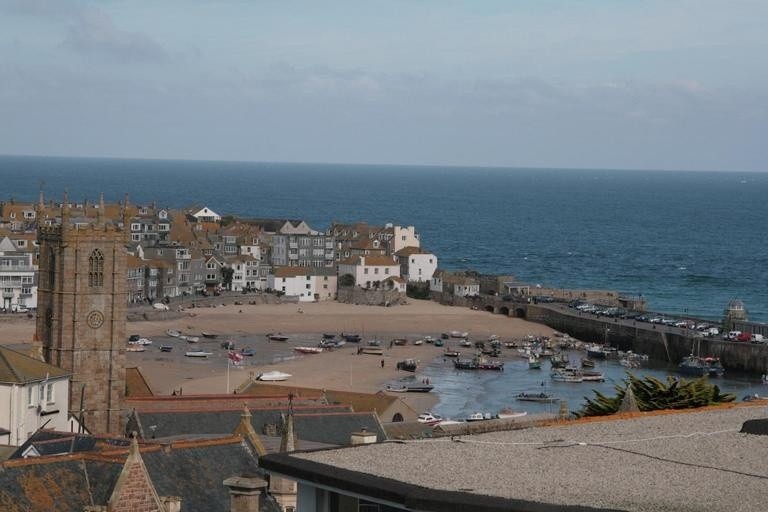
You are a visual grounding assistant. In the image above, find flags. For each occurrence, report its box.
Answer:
[228,350,243,362]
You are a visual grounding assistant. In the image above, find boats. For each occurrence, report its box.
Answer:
[257,370,292,381]
[742,394,767,402]
[394,331,504,372]
[417,412,443,423]
[221,340,257,356]
[466,408,528,422]
[269,334,290,341]
[168,328,219,343]
[127,334,153,352]
[294,332,384,354]
[386,374,434,392]
[504,323,648,382]
[160,344,174,352]
[184,351,214,357]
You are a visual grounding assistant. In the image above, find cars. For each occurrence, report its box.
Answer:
[487,289,555,303]
[568,301,768,344]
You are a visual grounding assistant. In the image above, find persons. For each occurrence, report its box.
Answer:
[426,377,429,385]
[381,359,384,369]
[397,362,400,371]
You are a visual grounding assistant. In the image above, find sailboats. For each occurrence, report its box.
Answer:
[677,337,726,377]
[512,380,560,403]
